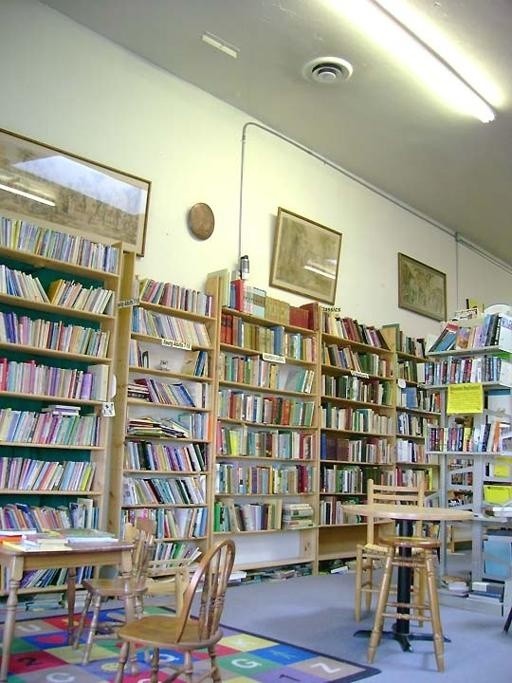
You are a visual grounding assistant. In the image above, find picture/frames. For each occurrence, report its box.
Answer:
[0,127,152,257]
[269,206,344,305]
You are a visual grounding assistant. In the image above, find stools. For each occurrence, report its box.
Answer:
[366,535,445,672]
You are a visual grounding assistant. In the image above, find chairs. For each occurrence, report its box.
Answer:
[73,515,237,683]
[355,478,430,626]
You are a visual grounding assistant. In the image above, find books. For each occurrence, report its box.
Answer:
[1,214,119,598]
[121,280,512,575]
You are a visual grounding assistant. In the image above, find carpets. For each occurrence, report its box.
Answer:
[0,605,384,683]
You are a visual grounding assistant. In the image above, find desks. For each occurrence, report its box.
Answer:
[341,501,475,653]
[0,529,140,682]
